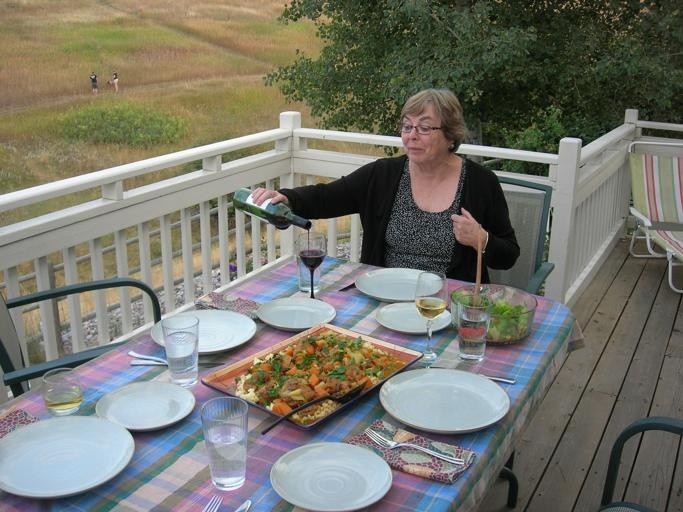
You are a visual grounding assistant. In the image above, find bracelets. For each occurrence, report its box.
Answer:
[482,231,489,253]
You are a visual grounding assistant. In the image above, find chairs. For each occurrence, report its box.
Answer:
[487,176,555,297]
[628,141,682,295]
[602,417,682,512]
[0,276,164,403]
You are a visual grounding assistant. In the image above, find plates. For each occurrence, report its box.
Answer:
[148,309,258,358]
[374,302,453,337]
[377,368,512,437]
[255,295,338,335]
[0,417,135,502]
[92,374,193,434]
[354,268,444,303]
[266,438,395,511]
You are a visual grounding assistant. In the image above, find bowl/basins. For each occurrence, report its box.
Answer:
[448,283,537,347]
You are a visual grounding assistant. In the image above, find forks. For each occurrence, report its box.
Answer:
[201,494,223,512]
[360,427,465,468]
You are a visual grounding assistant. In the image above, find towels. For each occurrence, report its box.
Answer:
[348,419,477,485]
[194,291,261,320]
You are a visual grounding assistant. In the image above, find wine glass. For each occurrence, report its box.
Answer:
[414,272,450,364]
[296,231,327,303]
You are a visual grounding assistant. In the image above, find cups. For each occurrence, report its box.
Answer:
[159,314,201,389]
[299,257,321,296]
[457,294,493,364]
[199,395,255,490]
[40,366,82,416]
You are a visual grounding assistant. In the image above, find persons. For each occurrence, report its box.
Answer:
[112,71,118,93]
[251,89,520,284]
[88,72,98,96]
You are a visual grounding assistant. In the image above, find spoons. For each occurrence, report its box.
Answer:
[260,379,371,436]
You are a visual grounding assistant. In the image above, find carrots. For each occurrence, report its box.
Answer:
[242,335,387,419]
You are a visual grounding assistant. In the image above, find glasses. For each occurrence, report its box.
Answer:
[402,122,441,135]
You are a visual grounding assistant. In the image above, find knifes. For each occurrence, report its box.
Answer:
[235,496,251,511]
[130,357,227,371]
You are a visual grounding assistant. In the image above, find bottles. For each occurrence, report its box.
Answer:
[232,186,312,232]
